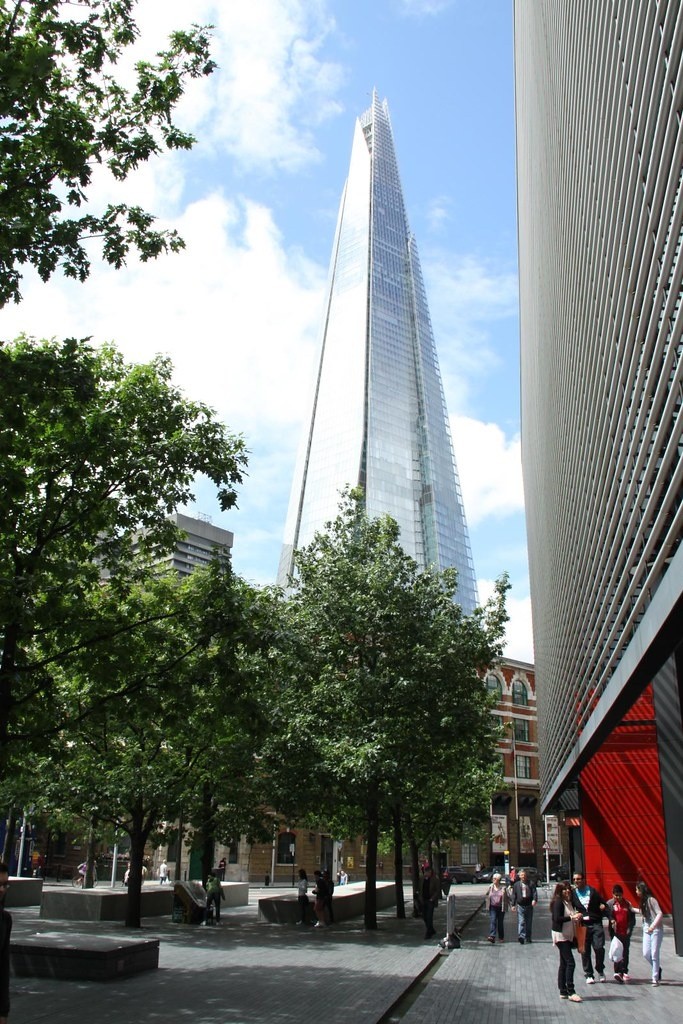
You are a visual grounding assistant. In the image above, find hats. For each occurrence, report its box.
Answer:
[163,859,167,864]
[492,873,502,880]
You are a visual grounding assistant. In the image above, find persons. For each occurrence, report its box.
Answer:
[200,873,225,926]
[123,864,148,886]
[569,870,609,984]
[475,861,485,873]
[549,881,583,1002]
[159,859,170,885]
[76,862,87,882]
[421,859,429,875]
[218,857,226,881]
[511,870,537,944]
[626,881,664,987]
[339,871,348,885]
[414,867,439,940]
[296,869,309,925]
[484,873,509,944]
[312,869,335,928]
[0,863,12,1024]
[604,884,636,983]
[509,865,520,886]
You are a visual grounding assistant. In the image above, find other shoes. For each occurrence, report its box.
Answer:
[525,939,531,944]
[313,920,327,928]
[200,920,207,926]
[296,920,303,926]
[651,982,660,987]
[518,937,524,944]
[499,937,504,943]
[488,936,495,943]
[658,967,662,980]
[431,928,436,936]
[424,929,432,939]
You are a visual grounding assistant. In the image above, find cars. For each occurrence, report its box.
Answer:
[473,866,505,883]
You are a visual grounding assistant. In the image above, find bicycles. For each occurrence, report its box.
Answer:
[71,873,97,889]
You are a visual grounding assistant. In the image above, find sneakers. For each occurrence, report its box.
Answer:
[586,976,595,984]
[599,975,606,982]
[567,993,583,1002]
[560,994,568,999]
[623,972,630,981]
[613,973,624,983]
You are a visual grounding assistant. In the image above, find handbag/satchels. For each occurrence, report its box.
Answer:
[575,919,587,955]
[608,935,624,963]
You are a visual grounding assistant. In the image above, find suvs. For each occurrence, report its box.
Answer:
[515,866,547,887]
[441,867,473,884]
[549,863,570,882]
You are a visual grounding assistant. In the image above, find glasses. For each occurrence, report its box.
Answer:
[0,881,8,888]
